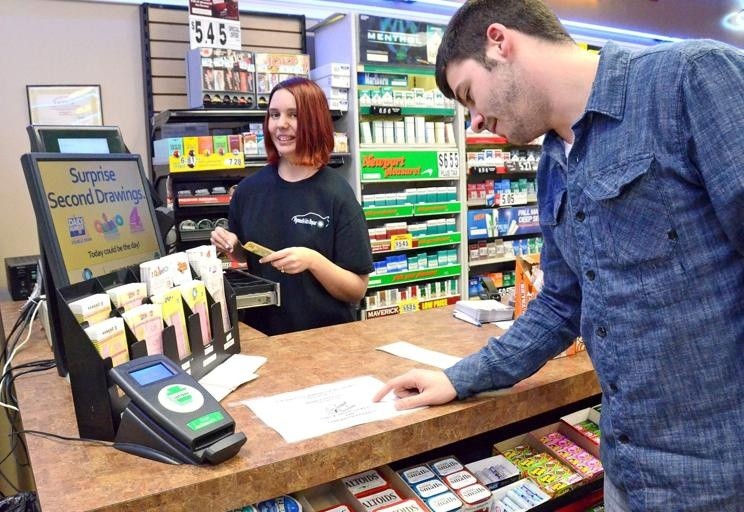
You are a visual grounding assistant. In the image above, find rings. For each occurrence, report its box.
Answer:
[281,265,285,274]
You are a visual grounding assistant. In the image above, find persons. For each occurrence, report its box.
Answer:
[371,0,744,512]
[210,79,376,335]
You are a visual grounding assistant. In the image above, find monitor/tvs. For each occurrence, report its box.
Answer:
[20,153,168,286]
[26,125,129,153]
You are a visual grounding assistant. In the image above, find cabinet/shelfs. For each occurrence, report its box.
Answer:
[455,19,601,304]
[311,8,466,321]
[138,2,351,257]
